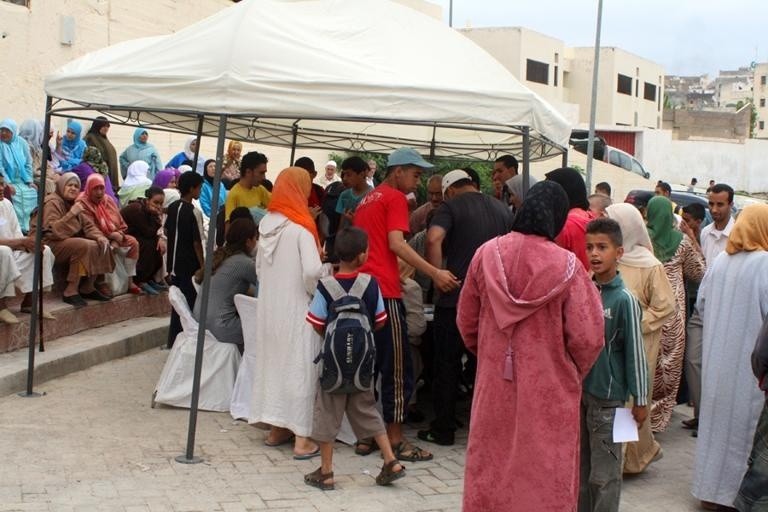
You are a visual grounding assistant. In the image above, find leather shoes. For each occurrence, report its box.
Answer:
[682,418,696,427]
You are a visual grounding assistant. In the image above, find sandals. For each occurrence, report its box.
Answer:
[376,460,406,485]
[304,467,334,490]
[355,438,379,456]
[382,440,434,461]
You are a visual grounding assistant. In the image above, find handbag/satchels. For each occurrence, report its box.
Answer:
[105,247,129,296]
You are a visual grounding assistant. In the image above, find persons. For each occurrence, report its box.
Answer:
[0,117,767,512]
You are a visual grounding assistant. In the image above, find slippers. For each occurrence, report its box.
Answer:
[83,289,110,301]
[62,294,86,306]
[0,309,21,324]
[265,432,294,446]
[21,305,55,320]
[293,443,321,459]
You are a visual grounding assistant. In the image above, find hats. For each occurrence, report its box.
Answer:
[387,146,434,169]
[441,169,472,202]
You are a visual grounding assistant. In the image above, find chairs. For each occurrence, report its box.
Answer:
[231,293,259,421]
[151,286,240,413]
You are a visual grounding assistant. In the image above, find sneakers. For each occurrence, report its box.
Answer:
[417,429,453,446]
[99,287,112,296]
[128,282,142,294]
[139,283,159,296]
[148,280,168,290]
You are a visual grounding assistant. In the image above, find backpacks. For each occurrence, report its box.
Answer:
[313,273,376,395]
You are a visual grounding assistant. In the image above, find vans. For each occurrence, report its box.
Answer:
[623,190,709,210]
[605,144,649,180]
